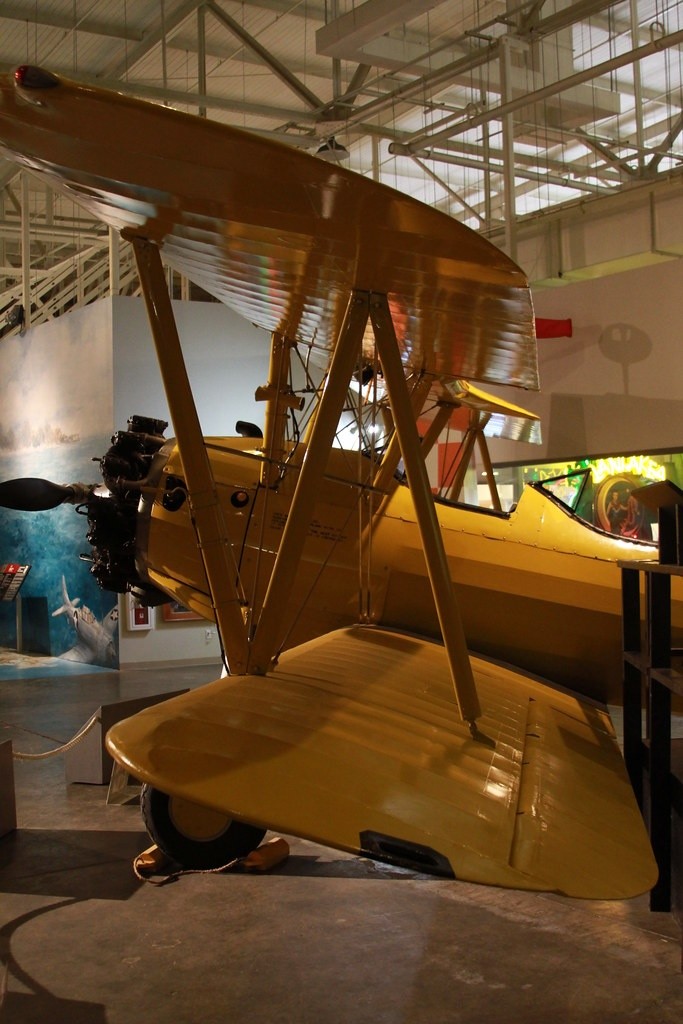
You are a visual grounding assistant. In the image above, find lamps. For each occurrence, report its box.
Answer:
[316,136,351,163]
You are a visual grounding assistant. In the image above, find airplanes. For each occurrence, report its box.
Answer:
[51,574,119,667]
[1,63,682,903]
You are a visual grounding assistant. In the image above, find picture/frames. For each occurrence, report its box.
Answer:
[162,602,204,620]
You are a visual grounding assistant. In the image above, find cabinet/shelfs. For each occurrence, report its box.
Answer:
[616,559,683,914]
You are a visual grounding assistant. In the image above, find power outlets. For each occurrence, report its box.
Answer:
[206,629,212,639]
